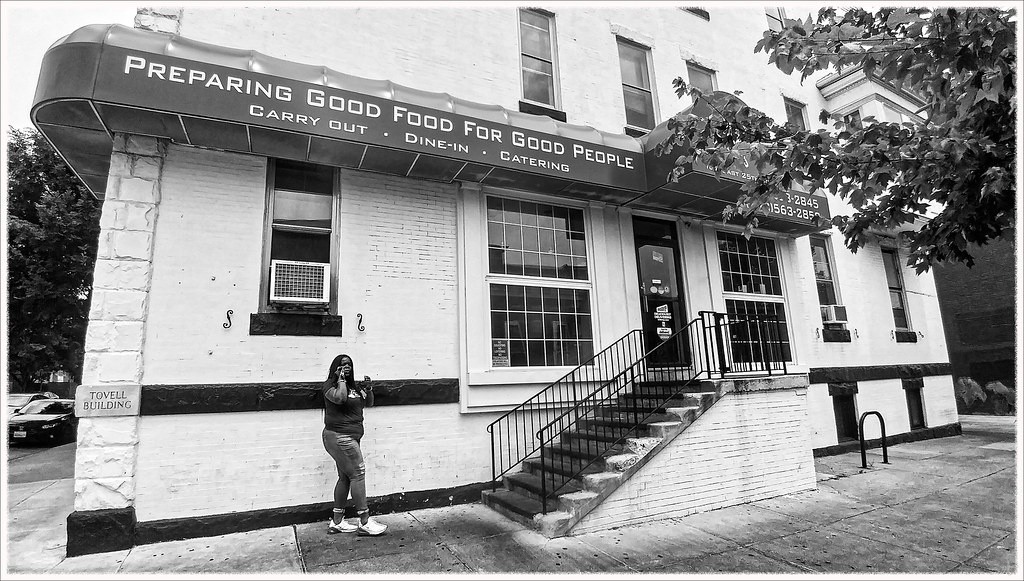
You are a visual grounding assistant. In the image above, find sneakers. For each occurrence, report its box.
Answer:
[357,518,388,535]
[328,515,358,533]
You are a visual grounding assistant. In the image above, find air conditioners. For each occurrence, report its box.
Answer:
[268,258,333,305]
[821,305,849,324]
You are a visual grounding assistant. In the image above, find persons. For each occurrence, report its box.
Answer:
[322,354,389,536]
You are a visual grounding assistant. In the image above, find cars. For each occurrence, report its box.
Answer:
[8,393,79,448]
[39,380,48,383]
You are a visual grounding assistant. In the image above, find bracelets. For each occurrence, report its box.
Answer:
[337,380,347,383]
[365,386,373,392]
